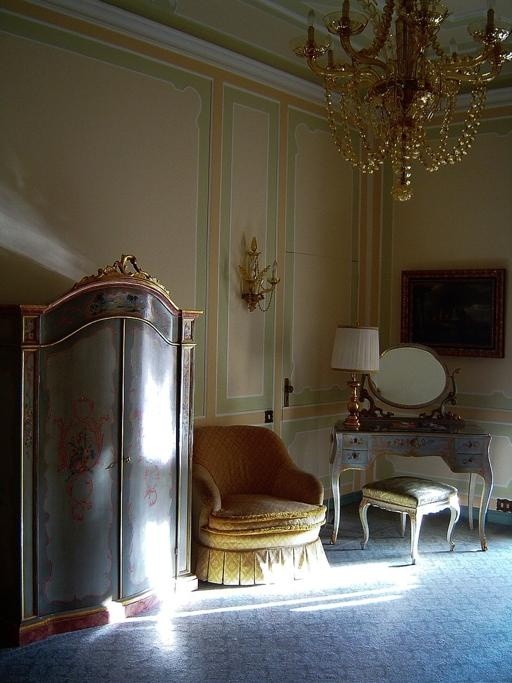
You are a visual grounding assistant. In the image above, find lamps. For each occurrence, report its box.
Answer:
[237,237,280,312]
[330,323,380,426]
[293,0,512,202]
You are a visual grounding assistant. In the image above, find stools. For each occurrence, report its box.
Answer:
[359,476,461,565]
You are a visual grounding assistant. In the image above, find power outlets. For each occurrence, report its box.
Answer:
[496,499,512,513]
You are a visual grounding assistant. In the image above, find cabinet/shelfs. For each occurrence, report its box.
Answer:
[0,254,204,649]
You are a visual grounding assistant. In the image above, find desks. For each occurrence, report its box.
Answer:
[329,420,493,552]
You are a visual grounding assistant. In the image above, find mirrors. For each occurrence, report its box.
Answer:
[358,343,463,427]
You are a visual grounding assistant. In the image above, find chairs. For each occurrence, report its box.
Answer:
[191,425,332,587]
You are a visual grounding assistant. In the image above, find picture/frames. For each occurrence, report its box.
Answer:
[400,268,507,358]
